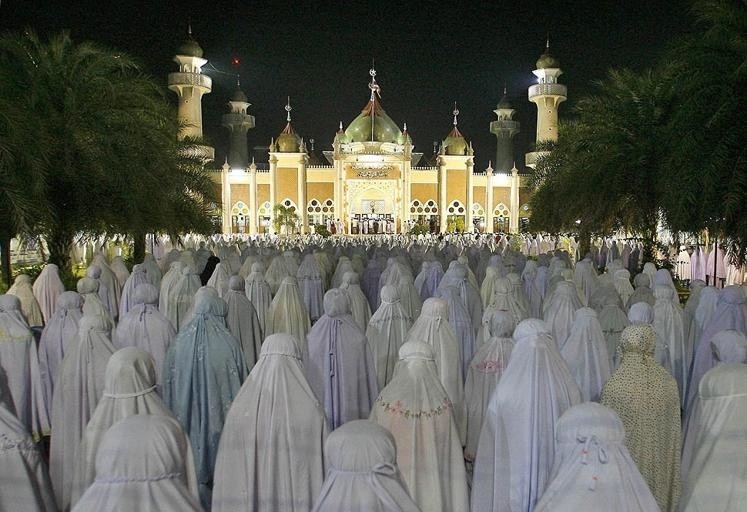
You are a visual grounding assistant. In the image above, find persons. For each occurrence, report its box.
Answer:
[0,212,747,510]
